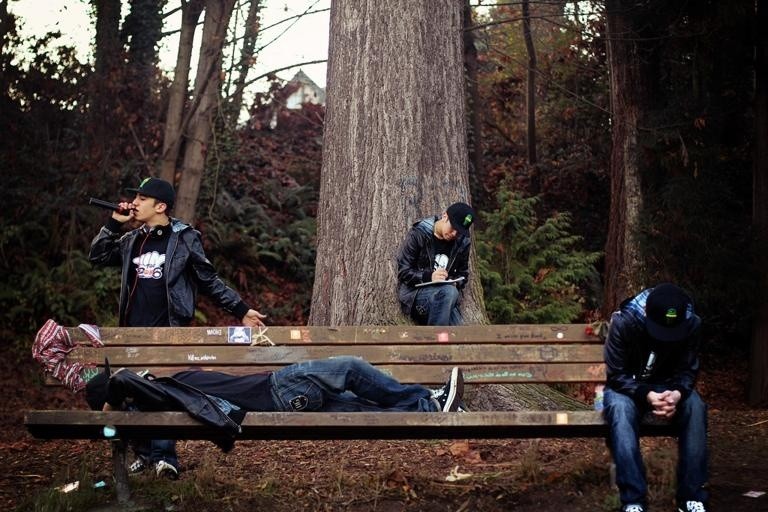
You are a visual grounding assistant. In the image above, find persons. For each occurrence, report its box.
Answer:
[87,176,270,481]
[396,202,479,327]
[84,356,466,426]
[601,281,709,512]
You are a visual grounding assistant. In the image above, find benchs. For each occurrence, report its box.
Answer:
[23,324,679,506]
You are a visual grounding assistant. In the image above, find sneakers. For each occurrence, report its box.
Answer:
[678,499,708,512]
[428,366,467,413]
[128,457,178,479]
[622,502,645,512]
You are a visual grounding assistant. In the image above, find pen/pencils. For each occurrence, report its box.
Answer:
[434,261,441,269]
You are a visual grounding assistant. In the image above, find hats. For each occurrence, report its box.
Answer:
[447,202,475,239]
[125,176,175,205]
[645,283,688,341]
[86,356,111,411]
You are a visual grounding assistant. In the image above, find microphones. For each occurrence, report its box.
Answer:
[88,197,130,216]
